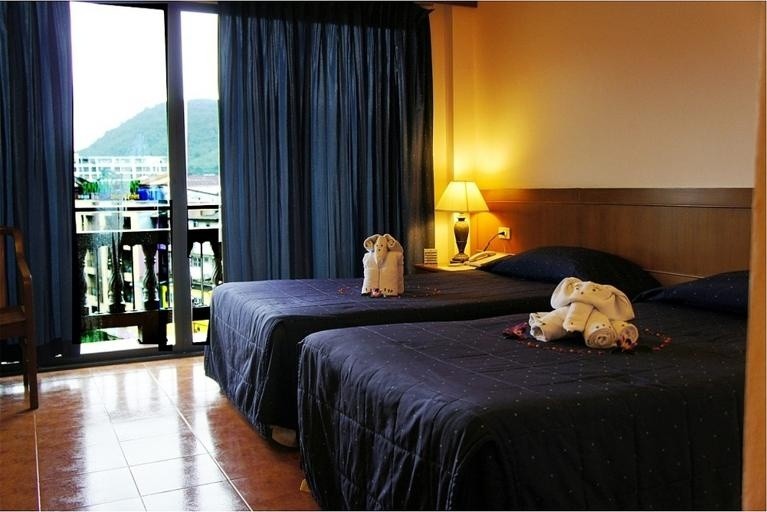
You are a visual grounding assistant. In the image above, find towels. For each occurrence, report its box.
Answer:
[361,233,406,299]
[527,275,639,348]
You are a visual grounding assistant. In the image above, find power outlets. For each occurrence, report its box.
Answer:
[498,226,510,239]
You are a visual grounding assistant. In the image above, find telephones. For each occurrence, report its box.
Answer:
[466,250,508,267]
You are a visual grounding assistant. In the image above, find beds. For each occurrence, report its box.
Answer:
[203,271,558,442]
[297,301,743,510]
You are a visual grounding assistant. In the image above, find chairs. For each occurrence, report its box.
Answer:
[0,225,39,411]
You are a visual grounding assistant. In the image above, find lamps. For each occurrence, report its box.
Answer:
[434,180,490,263]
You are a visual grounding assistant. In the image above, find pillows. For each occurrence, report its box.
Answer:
[476,246,657,291]
[633,269,747,315]
[472,244,749,320]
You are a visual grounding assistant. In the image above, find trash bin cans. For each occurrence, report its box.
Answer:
[138,310,166,343]
